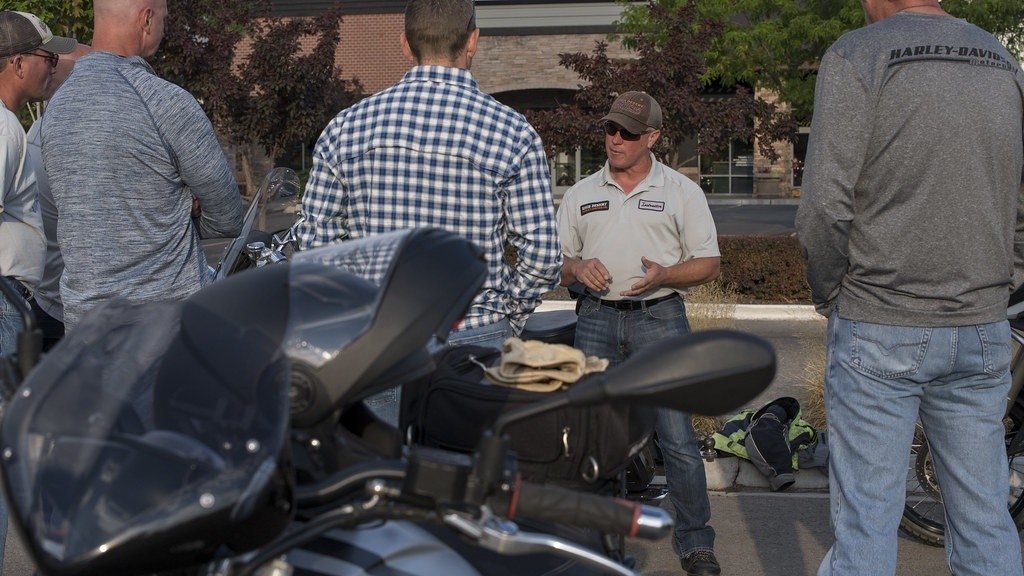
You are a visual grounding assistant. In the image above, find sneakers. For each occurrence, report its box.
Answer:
[681,551,721,575]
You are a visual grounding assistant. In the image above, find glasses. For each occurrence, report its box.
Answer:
[605,121,657,141]
[10,51,59,67]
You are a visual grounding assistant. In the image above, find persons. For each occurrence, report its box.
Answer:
[557,91,721,576]
[297,0,565,428]
[796,0,1024,576]
[0,0,244,576]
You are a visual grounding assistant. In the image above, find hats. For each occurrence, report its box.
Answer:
[597,91,661,134]
[0,9,78,57]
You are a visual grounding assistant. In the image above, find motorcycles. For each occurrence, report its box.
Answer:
[0,162,779,576]
[899,281,1024,548]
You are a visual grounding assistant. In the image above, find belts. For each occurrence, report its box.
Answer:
[588,291,680,311]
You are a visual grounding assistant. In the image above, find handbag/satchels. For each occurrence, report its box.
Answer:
[398,343,629,493]
